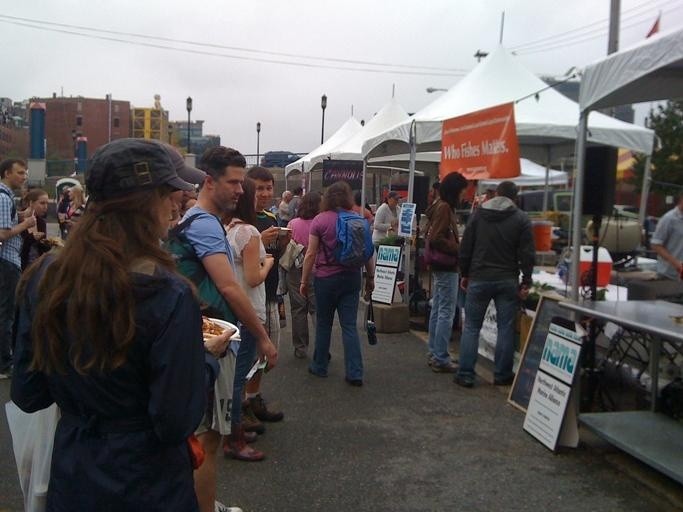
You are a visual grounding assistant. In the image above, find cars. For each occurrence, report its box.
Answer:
[611,202,664,231]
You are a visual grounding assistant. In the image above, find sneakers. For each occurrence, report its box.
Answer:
[451,372,476,388]
[344,375,363,387]
[307,364,328,378]
[213,499,243,512]
[431,357,457,373]
[246,393,284,423]
[427,351,458,366]
[492,370,517,386]
[237,399,266,436]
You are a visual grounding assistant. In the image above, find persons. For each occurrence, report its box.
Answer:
[1,139,403,512]
[424,172,472,374]
[452,181,536,387]
[650,190,683,282]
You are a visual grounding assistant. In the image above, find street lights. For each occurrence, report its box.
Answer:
[69,128,78,177]
[256,123,264,168]
[318,92,331,147]
[423,84,450,97]
[186,93,194,155]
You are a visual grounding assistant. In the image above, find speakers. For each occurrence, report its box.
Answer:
[582,146,618,217]
[412,176,430,214]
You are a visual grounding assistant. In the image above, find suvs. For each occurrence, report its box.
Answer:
[260,148,303,171]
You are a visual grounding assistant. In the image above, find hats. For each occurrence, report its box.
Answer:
[151,138,208,185]
[386,191,403,201]
[82,137,195,203]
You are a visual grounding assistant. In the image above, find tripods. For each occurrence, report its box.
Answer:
[410,214,427,316]
[580,216,617,412]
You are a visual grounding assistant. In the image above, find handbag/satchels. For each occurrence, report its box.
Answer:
[422,236,460,271]
[277,239,305,273]
[159,212,239,327]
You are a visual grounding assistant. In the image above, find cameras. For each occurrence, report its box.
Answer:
[367,321,377,345]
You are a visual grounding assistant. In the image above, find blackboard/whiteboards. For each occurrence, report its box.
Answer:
[507,294,577,414]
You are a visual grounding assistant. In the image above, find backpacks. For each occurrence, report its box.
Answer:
[327,206,375,269]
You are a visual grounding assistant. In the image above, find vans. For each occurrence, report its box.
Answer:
[516,188,593,242]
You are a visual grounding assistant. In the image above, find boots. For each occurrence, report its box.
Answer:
[222,422,265,461]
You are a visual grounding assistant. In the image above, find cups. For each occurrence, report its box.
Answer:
[274,227,290,235]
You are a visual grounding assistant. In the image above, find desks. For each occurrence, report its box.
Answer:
[558,299,683,483]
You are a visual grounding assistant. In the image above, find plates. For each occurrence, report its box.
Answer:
[203,317,241,340]
